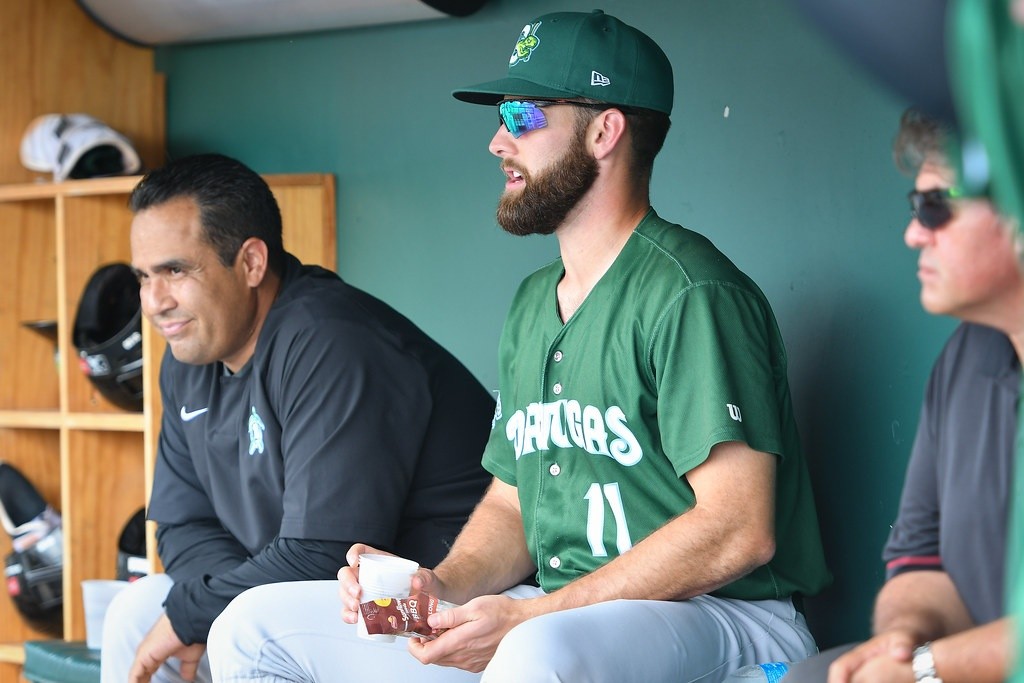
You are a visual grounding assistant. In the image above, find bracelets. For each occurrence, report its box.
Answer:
[911,642,943,683]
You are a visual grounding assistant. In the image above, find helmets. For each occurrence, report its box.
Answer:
[70,261,143,413]
[5,548,63,637]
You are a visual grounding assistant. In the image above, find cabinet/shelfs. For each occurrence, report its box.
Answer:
[0,173,337,683]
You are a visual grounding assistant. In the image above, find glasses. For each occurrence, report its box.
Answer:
[908,188,988,230]
[498,99,607,139]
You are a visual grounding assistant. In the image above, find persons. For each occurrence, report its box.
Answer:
[200,11,823,683]
[97,150,545,683]
[779,81,1024,683]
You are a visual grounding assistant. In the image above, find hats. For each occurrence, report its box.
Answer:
[452,9,674,115]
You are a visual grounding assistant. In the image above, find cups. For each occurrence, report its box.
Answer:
[355,554,419,643]
[81,579,130,649]
